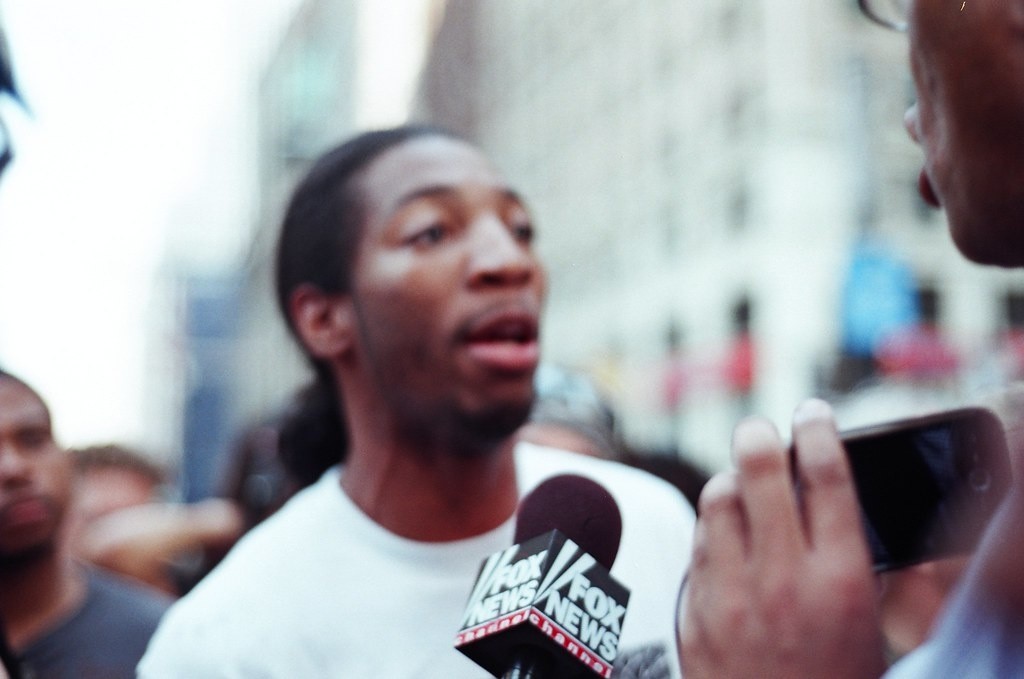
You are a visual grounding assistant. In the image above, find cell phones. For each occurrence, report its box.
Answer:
[788,405,1012,580]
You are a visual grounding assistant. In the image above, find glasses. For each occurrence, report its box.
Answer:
[858,0,913,34]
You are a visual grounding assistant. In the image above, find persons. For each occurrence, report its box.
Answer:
[56,376,971,658]
[686,0,1022,676]
[140,128,703,679]
[0,374,185,679]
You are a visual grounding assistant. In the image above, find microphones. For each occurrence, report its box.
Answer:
[455,473,630,678]
